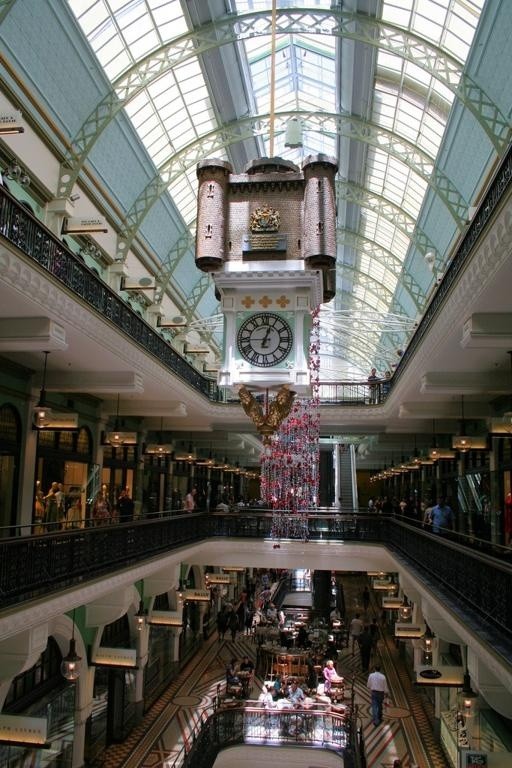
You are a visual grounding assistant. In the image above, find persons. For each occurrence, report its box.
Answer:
[368,368,380,403]
[383,370,391,397]
[33,476,198,535]
[391,349,404,368]
[213,569,391,738]
[333,496,343,509]
[366,492,457,547]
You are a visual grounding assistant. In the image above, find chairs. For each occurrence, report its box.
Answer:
[208,607,348,714]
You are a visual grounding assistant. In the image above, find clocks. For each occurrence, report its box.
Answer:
[237,311,294,368]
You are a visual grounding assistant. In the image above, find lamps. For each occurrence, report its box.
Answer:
[61,609,85,682]
[28,351,53,429]
[201,566,212,588]
[398,591,414,620]
[455,392,475,456]
[454,642,482,719]
[376,568,399,595]
[366,417,446,484]
[176,562,186,603]
[105,392,127,448]
[134,579,146,632]
[418,621,436,653]
[152,415,264,482]
